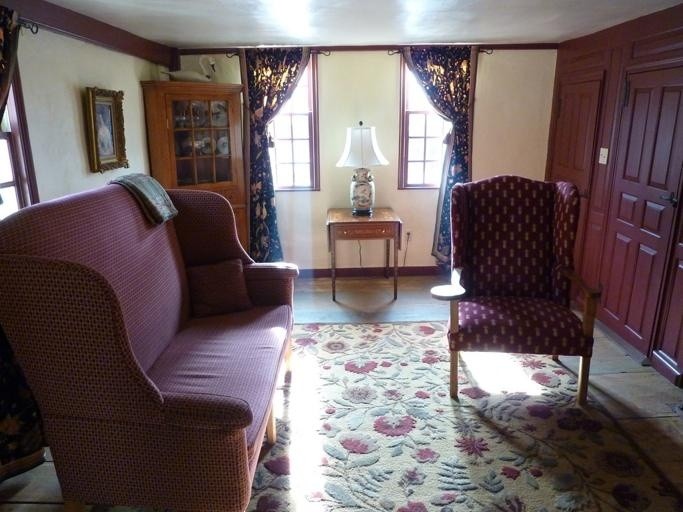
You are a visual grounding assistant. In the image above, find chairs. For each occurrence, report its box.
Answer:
[430,173,599,405]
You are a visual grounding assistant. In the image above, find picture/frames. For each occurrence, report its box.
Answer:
[85,86,128,173]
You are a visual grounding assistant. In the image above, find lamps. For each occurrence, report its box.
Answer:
[335,120,390,217]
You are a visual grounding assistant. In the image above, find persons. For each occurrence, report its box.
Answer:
[97,112,114,156]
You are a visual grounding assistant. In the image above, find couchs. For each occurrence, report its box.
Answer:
[0,182,300,512]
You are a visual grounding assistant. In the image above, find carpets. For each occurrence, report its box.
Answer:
[91,319,683,512]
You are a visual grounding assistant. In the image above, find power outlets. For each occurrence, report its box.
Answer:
[406,228,413,241]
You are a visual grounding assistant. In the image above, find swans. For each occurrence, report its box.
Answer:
[161,54,216,82]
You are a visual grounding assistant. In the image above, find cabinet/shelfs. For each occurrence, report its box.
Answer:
[139,79,249,255]
[326,206,403,301]
